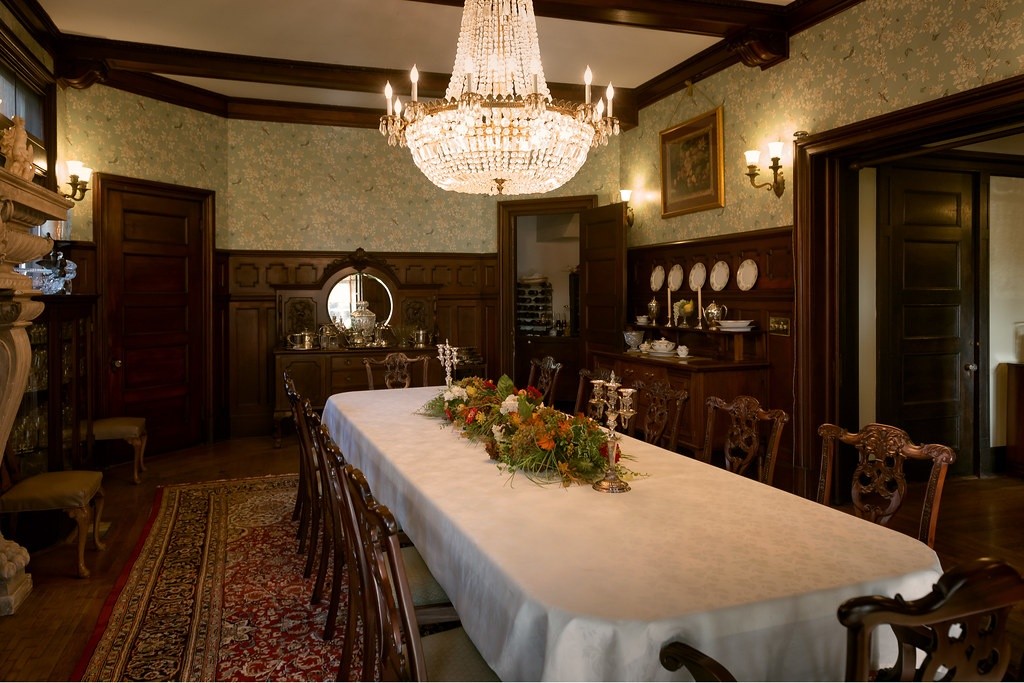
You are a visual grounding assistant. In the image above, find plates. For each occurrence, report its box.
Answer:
[667,264,684,292]
[737,258,757,291]
[649,265,665,291]
[688,263,705,292]
[646,350,694,359]
[635,321,650,326]
[710,261,729,292]
[716,320,752,334]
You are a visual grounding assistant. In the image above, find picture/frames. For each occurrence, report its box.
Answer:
[659,105,726,220]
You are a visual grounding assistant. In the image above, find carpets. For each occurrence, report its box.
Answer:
[78,468,386,683]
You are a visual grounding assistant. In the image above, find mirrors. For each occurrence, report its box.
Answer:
[327,272,394,333]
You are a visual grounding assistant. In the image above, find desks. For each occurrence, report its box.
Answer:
[321,384,947,683]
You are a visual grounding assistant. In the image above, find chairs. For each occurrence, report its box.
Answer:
[524,355,561,410]
[573,368,622,429]
[814,420,955,552]
[700,395,791,486]
[657,555,1024,683]
[626,378,690,454]
[280,370,504,682]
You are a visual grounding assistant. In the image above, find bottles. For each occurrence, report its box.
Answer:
[352,300,376,340]
[556,314,561,335]
[561,314,566,335]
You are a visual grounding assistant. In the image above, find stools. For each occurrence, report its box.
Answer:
[92,417,149,484]
[0,470,108,580]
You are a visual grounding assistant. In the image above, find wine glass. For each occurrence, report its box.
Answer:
[388,322,419,348]
[551,319,557,337]
[623,331,645,352]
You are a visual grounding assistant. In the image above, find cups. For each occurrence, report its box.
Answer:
[541,314,546,323]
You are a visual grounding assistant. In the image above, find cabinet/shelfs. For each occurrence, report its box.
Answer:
[515,335,579,401]
[411,350,446,388]
[273,354,325,412]
[325,351,411,409]
[589,348,768,463]
[0,293,100,555]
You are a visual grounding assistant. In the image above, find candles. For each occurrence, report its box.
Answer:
[667,287,671,316]
[697,286,702,318]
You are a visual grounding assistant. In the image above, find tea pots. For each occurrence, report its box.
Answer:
[649,296,728,331]
[287,326,433,351]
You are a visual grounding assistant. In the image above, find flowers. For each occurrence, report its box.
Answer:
[411,373,651,493]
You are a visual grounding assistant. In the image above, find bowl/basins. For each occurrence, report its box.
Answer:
[636,315,649,323]
[640,337,689,357]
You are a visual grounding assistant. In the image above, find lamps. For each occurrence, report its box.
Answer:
[379,0,621,196]
[618,189,635,228]
[66,167,93,202]
[56,159,84,198]
[767,141,785,199]
[744,150,773,192]
